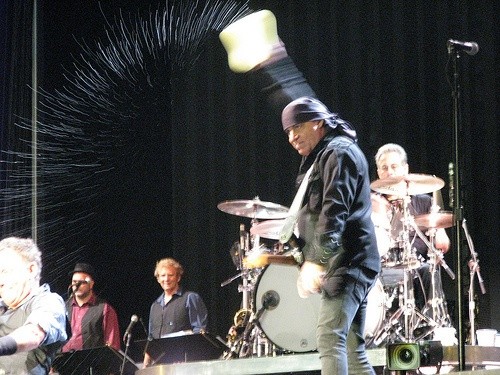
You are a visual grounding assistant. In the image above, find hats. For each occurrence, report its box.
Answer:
[68,263,98,277]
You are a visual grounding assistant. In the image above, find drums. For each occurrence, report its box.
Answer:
[253,246,386,356]
[381,246,423,287]
[369,192,393,258]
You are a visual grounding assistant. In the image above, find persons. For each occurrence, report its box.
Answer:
[264,41,381,375]
[49,262,121,374]
[142,258,208,369]
[0,237,72,375]
[363,142,457,346]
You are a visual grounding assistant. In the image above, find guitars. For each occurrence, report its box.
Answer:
[244,231,307,272]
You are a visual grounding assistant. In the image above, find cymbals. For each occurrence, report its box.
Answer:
[409,213,466,229]
[218,199,301,240]
[371,172,445,195]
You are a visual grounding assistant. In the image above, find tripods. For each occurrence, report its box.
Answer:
[217,213,287,359]
[365,188,460,348]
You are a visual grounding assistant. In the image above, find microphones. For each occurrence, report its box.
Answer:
[448,39,479,55]
[124,315,139,340]
[75,277,91,286]
